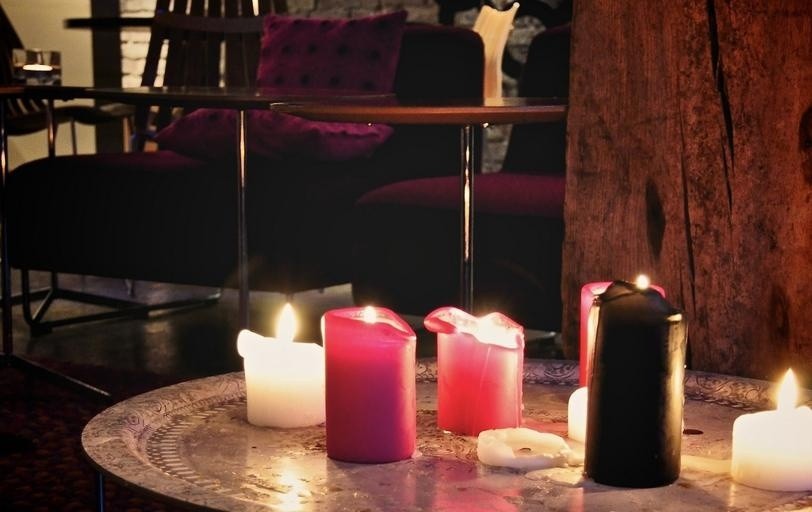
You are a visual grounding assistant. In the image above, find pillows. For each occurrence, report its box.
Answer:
[153,105,393,159]
[259,16,402,91]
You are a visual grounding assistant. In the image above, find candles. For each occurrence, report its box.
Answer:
[579,279,669,387]
[424,304,529,436]
[476,428,579,468]
[565,386,589,441]
[730,365,812,494]
[319,305,419,463]
[235,304,325,430]
[584,279,689,489]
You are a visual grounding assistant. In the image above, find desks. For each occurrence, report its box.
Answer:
[272,92,576,311]
[89,76,396,334]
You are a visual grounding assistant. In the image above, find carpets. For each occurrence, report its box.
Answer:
[2,358,205,511]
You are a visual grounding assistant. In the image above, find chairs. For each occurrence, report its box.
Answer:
[1,8,139,165]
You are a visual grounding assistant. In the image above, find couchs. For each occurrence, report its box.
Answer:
[3,22,568,335]
